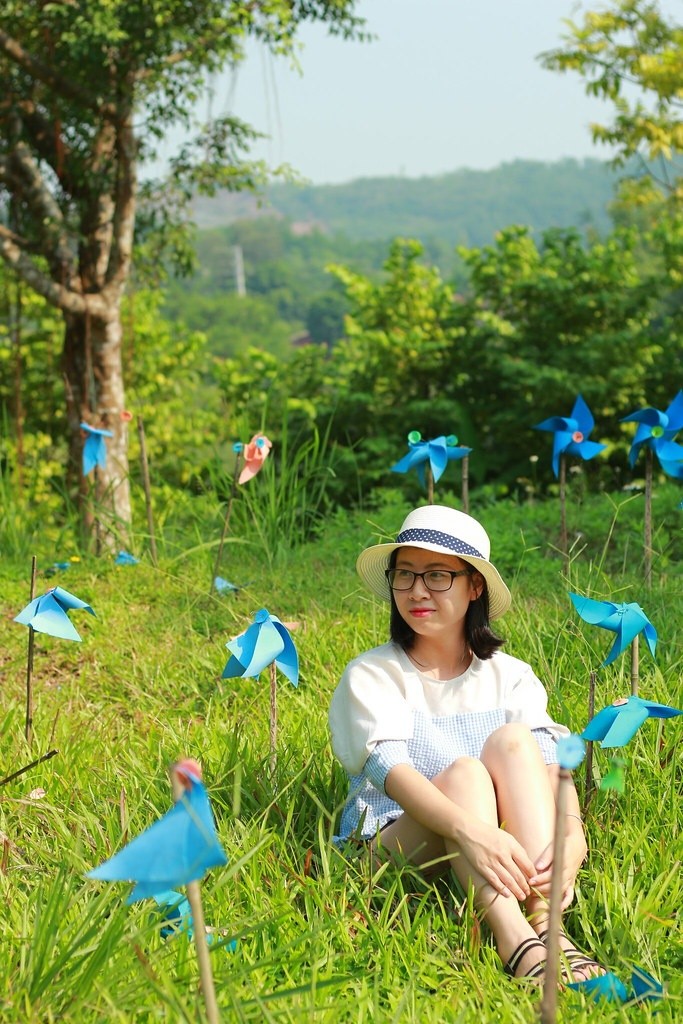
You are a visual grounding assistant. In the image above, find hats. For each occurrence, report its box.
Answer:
[356,505,512,620]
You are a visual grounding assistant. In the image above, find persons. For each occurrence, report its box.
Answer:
[323,505,609,985]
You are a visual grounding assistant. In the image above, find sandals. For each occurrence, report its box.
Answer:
[539,928,606,985]
[503,938,574,995]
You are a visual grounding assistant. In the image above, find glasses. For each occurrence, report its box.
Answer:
[384,566,467,591]
